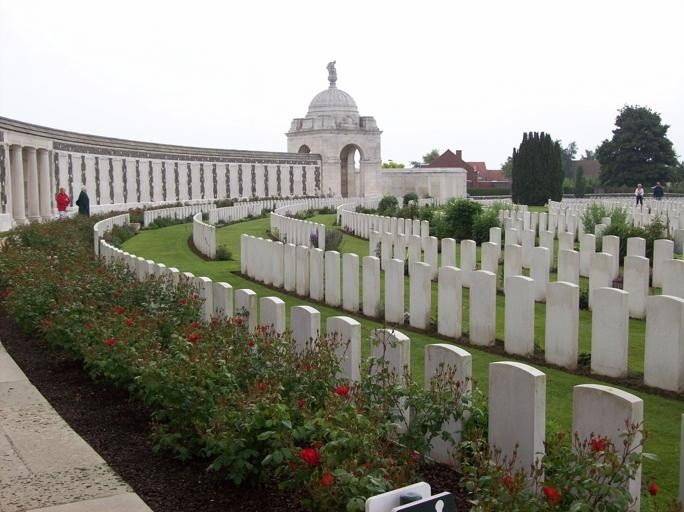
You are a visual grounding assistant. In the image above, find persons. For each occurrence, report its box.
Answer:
[654,182,663,200]
[75,185,90,217]
[635,183,644,213]
[56,187,70,218]
